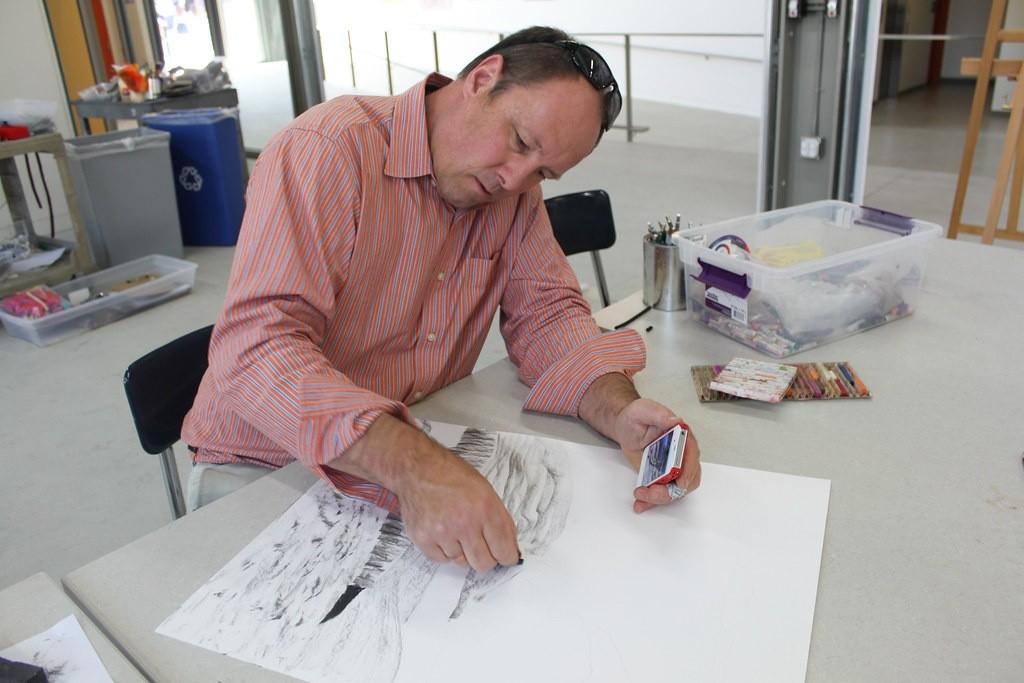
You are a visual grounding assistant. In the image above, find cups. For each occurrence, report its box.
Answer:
[0,220,30,262]
[643,234,705,311]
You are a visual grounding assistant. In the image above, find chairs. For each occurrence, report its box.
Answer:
[120,325,219,517]
[542,190,619,309]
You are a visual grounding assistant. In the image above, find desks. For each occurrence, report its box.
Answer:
[0,130,96,301]
[0,571,150,683]
[55,236,1023,682]
[71,88,250,194]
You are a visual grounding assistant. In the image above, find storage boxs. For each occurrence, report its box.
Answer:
[0,253,198,348]
[672,199,942,360]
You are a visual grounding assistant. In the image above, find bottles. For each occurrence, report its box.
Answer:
[118,77,129,100]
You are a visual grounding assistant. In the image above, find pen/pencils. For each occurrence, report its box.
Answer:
[645,213,703,247]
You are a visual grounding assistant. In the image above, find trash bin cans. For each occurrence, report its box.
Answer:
[142,106,248,248]
[63,125,185,272]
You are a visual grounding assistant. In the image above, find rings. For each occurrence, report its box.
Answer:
[452,555,466,566]
[668,483,686,500]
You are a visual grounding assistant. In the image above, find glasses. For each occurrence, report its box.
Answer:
[487,39,624,133]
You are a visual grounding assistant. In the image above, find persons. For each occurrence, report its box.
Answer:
[180,26,701,578]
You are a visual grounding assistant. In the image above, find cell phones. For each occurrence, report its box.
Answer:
[637,423,688,488]
